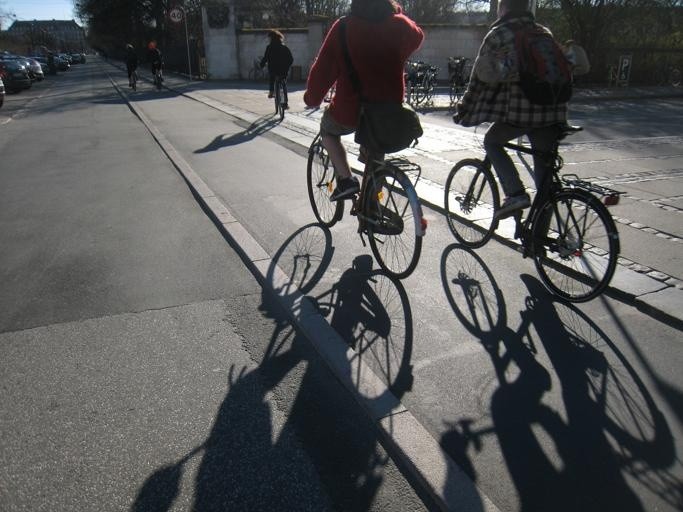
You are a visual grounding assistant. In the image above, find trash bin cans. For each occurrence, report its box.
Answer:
[291,66,300,81]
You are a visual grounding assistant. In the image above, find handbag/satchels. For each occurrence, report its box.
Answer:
[352,96,425,154]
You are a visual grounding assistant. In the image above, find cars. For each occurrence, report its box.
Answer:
[0,51,85,108]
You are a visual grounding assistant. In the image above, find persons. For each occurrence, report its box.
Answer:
[563,39,591,84]
[302,1,424,235]
[260,30,294,110]
[122,43,138,87]
[146,41,164,84]
[453,0,569,256]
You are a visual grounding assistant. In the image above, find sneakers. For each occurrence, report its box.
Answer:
[326,173,361,203]
[493,191,533,221]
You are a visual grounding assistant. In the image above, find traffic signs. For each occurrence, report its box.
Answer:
[168,9,183,23]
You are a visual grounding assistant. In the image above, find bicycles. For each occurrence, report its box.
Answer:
[249,61,264,83]
[404,57,471,102]
[272,76,288,121]
[445,112,627,303]
[304,99,427,280]
[129,62,164,91]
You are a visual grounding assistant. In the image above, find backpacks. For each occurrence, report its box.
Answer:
[499,19,574,107]
